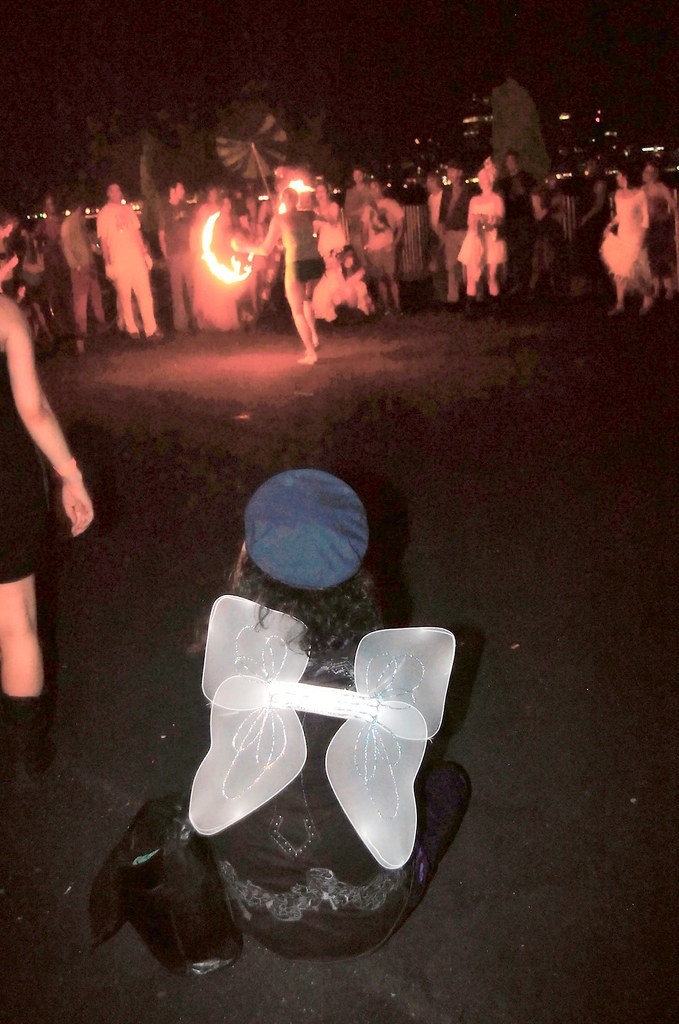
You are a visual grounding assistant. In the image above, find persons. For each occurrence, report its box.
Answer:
[0,213,95,775]
[189,470,470,963]
[0,152,678,367]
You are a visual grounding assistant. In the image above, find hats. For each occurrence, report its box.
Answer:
[244,468,370,589]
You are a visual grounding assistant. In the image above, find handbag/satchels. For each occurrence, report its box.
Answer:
[90,796,242,978]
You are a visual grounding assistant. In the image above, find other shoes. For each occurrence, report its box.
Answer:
[639,302,654,318]
[608,306,625,317]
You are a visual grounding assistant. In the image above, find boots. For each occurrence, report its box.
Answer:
[0,685,56,780]
